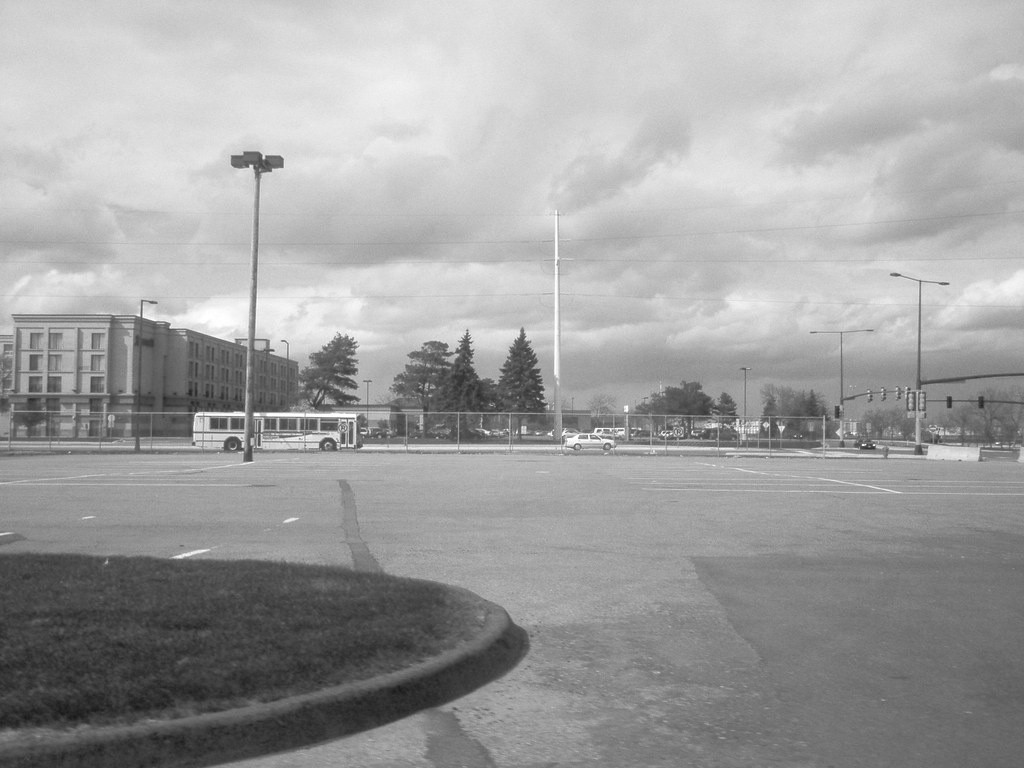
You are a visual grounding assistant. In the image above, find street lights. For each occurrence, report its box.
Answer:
[230,151,283,462]
[890,272,949,455]
[134,299,158,453]
[810,329,874,447]
[741,367,751,424]
[363,379,372,427]
[281,339,289,411]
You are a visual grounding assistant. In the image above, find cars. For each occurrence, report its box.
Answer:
[854,438,877,450]
[360,427,397,440]
[564,433,617,450]
[594,426,740,440]
[408,424,516,439]
[535,427,580,438]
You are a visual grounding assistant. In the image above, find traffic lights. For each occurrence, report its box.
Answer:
[835,406,839,418]
[978,395,984,408]
[947,395,952,408]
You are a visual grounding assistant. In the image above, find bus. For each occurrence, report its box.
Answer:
[191,411,365,452]
